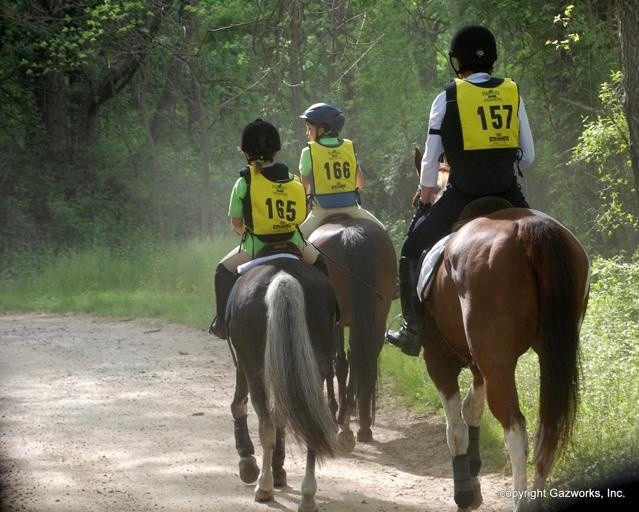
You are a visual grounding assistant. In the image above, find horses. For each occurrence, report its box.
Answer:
[413,145,592,512]
[223,255,340,512]
[301,218,400,459]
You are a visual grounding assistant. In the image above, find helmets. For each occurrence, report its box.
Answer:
[242,118,282,155]
[299,102,345,132]
[448,26,499,61]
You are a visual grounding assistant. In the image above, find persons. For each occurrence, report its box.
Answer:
[297,101,387,242]
[201,118,342,339]
[384,25,538,358]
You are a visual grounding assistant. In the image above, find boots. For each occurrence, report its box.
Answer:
[383,257,423,357]
[211,264,234,338]
